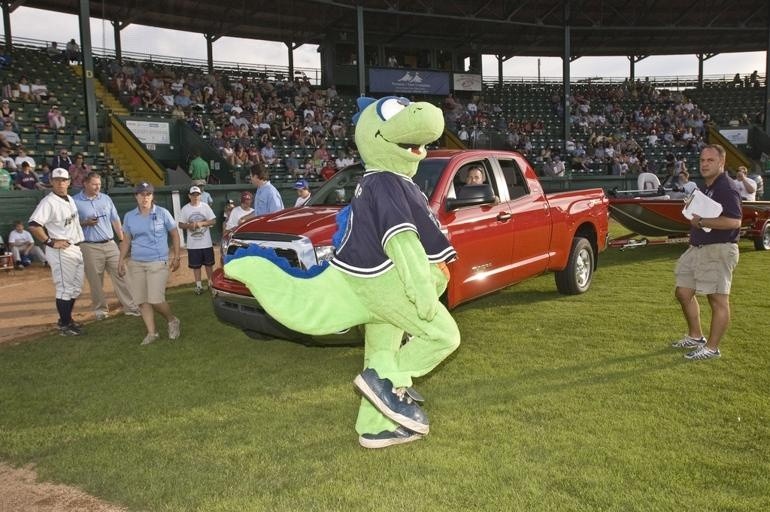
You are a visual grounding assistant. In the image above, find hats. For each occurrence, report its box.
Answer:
[240,191,252,200]
[49,167,70,179]
[292,180,309,191]
[197,180,206,185]
[189,186,201,194]
[133,181,154,193]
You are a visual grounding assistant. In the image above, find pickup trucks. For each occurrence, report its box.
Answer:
[206,147,610,349]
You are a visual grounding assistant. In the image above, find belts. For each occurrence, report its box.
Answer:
[83,239,113,244]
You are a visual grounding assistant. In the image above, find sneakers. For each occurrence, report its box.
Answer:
[16,265,24,269]
[141,333,160,345]
[96,311,109,320]
[167,317,181,341]
[57,318,85,336]
[682,346,722,361]
[672,336,707,348]
[41,261,49,266]
[125,308,142,316]
[193,286,203,295]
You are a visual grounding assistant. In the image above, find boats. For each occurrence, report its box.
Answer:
[605,189,768,250]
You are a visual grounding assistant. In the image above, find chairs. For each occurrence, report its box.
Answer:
[0,44,770,197]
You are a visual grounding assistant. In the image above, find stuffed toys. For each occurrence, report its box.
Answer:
[221,95,461,448]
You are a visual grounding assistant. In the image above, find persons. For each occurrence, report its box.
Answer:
[565,74,710,174]
[739,110,766,163]
[2,39,354,189]
[445,82,567,177]
[2,164,315,347]
[670,144,743,360]
[733,72,764,87]
[659,151,757,203]
